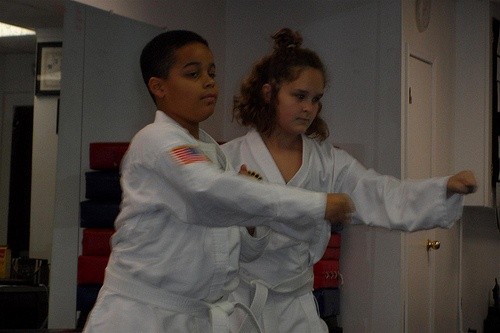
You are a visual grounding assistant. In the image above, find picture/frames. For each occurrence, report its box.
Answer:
[36,40,63,98]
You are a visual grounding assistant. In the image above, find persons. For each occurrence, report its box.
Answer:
[213,27,479,332]
[82,29,354,333]
